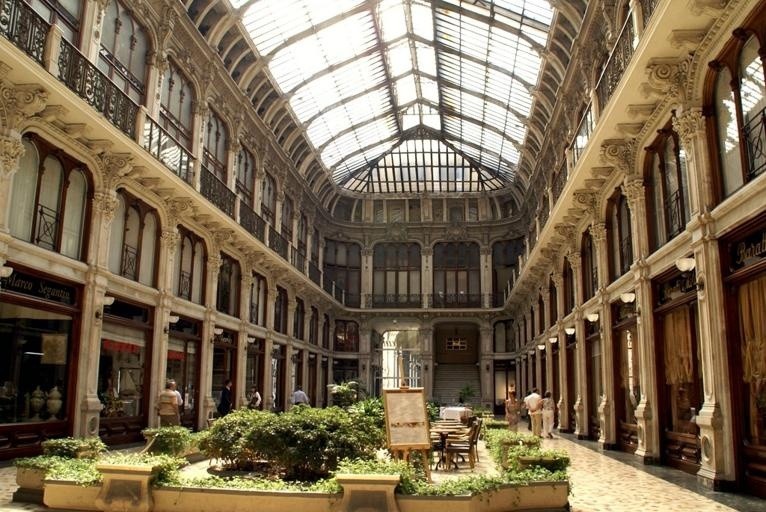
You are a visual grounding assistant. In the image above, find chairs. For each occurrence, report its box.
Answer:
[429,414,483,470]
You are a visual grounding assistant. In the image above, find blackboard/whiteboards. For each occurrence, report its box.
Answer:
[383,388,431,450]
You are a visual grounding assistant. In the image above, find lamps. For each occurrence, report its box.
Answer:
[93,295,328,366]
[675,256,705,294]
[507,292,641,366]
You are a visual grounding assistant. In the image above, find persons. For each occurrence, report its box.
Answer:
[154,377,183,425]
[217,380,233,416]
[505,388,555,439]
[246,386,262,411]
[290,384,311,414]
